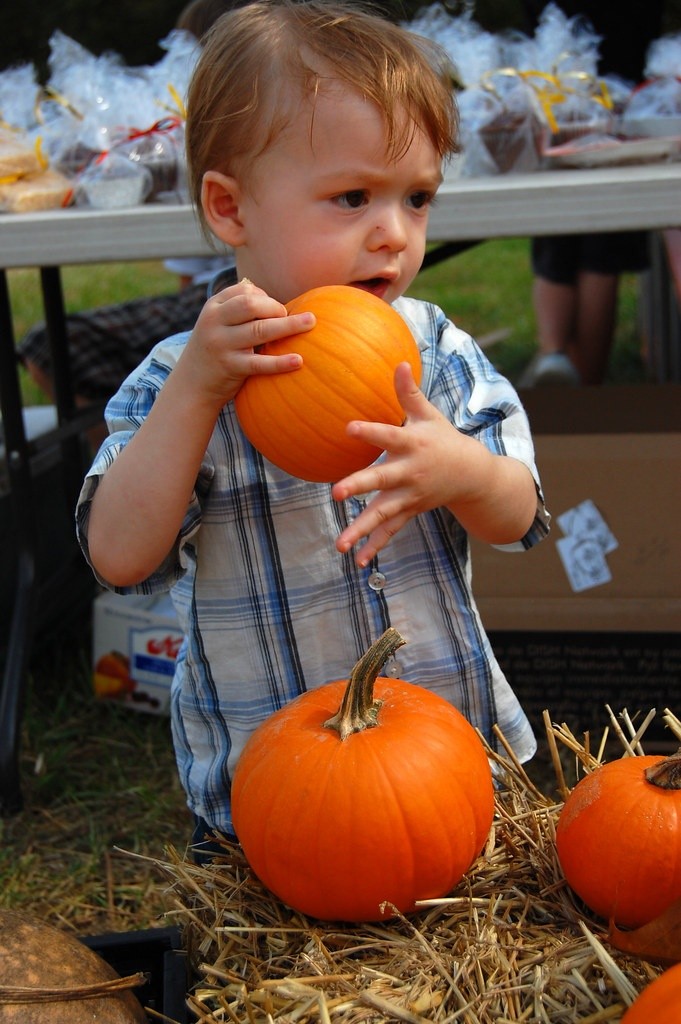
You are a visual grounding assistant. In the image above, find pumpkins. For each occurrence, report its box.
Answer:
[556,748,681,928]
[619,961,681,1023]
[233,629,495,922]
[235,284,423,483]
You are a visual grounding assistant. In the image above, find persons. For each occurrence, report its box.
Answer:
[74,1,551,865]
[15,0,680,408]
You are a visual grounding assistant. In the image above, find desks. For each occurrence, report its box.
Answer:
[0,161,681,817]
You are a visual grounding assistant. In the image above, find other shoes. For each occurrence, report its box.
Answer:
[532,352,578,391]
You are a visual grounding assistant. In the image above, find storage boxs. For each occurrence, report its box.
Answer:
[93,590,184,716]
[469,381,681,631]
